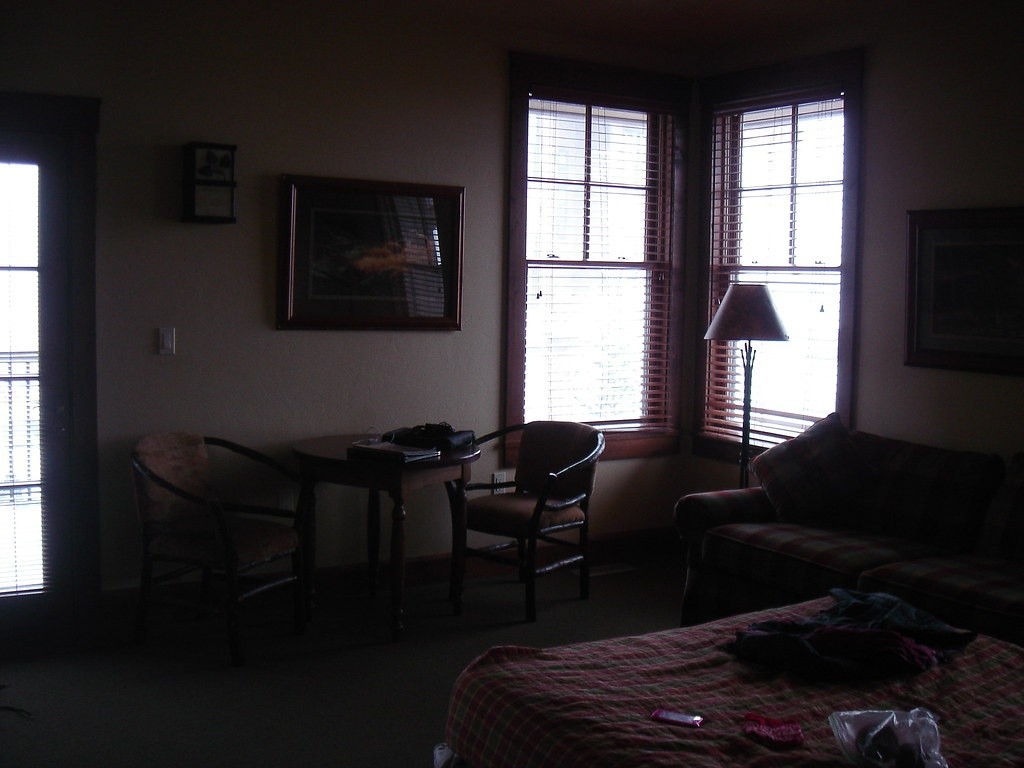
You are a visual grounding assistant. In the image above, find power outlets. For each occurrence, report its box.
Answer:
[492,472,507,494]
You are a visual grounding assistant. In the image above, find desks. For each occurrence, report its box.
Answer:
[290,433,481,644]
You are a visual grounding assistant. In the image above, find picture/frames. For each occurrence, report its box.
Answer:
[275,172,466,331]
[903,206,1024,377]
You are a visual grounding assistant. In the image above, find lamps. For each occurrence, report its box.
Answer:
[703,281,789,489]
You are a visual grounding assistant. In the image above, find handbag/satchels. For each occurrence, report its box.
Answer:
[382,428,476,450]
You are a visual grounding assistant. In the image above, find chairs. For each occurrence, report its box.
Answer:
[444,421,606,623]
[129,432,310,668]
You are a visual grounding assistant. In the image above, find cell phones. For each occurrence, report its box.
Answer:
[651,709,704,728]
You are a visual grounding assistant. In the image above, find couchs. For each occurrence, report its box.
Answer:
[672,428,1023,647]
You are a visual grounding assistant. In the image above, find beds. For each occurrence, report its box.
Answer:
[444,588,1024,768]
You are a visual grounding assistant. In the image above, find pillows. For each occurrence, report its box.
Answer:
[746,412,885,522]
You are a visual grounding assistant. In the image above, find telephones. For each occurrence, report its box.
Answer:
[383,423,475,452]
